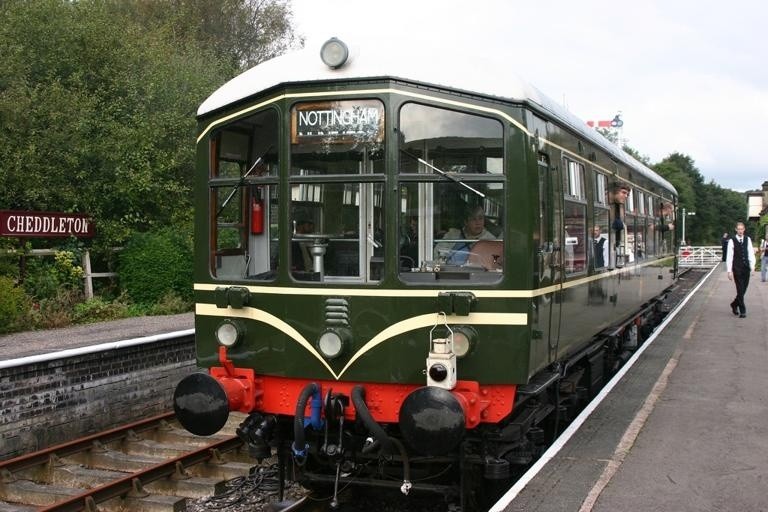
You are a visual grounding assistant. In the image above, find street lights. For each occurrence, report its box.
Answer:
[681,208,696,246]
[611,114,623,148]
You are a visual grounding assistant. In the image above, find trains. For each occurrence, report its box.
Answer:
[173,37,678,511]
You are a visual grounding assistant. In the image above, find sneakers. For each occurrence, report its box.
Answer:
[731,302,746,318]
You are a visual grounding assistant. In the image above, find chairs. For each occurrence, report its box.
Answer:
[274,200,506,268]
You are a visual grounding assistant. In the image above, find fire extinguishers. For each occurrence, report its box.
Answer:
[252,184,264,235]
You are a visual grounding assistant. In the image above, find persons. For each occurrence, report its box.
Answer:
[721,232,730,262]
[272,213,318,273]
[725,222,756,317]
[636,232,646,260]
[409,216,419,242]
[429,201,495,268]
[612,183,632,249]
[661,203,676,231]
[545,239,570,281]
[759,233,768,282]
[586,223,618,309]
